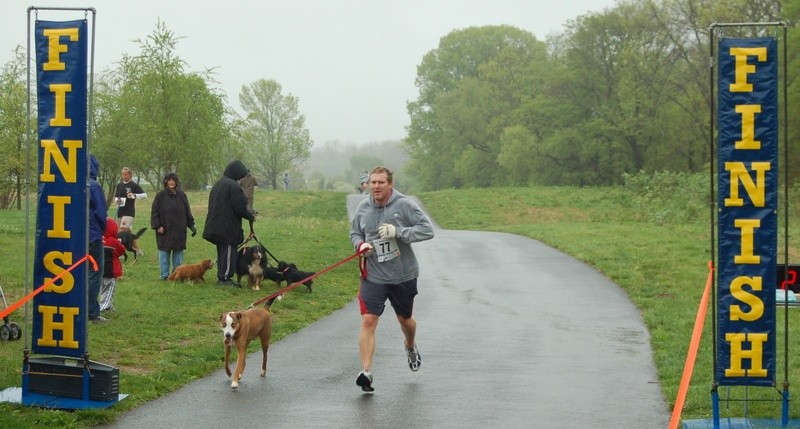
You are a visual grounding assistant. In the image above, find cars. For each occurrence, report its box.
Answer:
[131,177,152,185]
[201,185,213,191]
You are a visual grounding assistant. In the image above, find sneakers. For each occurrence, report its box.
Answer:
[356,370,374,391]
[404,339,421,371]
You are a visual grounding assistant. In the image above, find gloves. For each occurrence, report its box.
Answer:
[360,243,373,257]
[191,227,196,237]
[378,223,395,240]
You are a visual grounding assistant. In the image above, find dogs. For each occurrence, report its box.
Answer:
[219,292,283,389]
[168,259,214,287]
[117,227,148,261]
[278,261,316,294]
[236,244,267,290]
[264,263,297,287]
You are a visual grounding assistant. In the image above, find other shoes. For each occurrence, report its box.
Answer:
[92,316,109,324]
[138,249,144,257]
[216,280,242,289]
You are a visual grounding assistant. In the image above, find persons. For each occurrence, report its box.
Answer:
[114,167,147,259]
[284,173,289,190]
[88,155,108,321]
[151,172,197,280]
[99,219,125,312]
[201,159,258,287]
[349,166,435,392]
